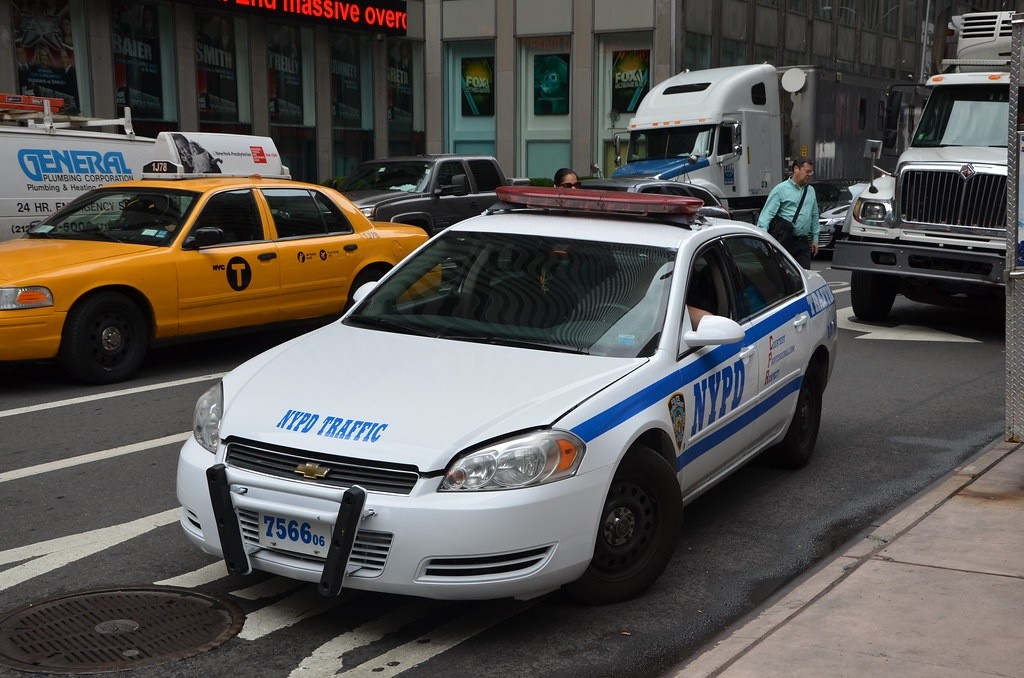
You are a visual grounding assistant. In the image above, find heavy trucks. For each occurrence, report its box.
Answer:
[830,6,1024,325]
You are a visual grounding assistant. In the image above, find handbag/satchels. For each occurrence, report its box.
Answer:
[767,214,792,245]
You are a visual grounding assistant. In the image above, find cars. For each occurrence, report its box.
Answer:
[176,183,839,612]
[2,174,428,387]
[807,204,851,259]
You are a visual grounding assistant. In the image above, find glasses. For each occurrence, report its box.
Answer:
[557,182,581,189]
[800,166,815,176]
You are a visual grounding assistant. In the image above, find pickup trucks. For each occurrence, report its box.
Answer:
[331,153,529,237]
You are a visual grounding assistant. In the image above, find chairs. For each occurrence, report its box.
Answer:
[116,194,179,231]
[479,250,552,327]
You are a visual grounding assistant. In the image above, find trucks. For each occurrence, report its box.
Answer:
[590,60,930,235]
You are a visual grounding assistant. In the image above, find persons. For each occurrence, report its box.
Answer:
[629,260,717,333]
[554,168,582,190]
[172,134,224,174]
[757,156,820,269]
[15,0,411,121]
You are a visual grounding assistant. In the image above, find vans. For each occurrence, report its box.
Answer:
[577,177,734,219]
